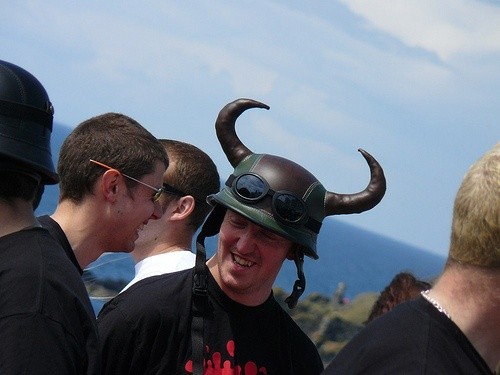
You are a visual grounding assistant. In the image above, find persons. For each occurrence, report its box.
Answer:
[322,140,500,375]
[361,272,431,326]
[97,98,386,375]
[0,60,101,375]
[36,113,169,276]
[119,139,220,294]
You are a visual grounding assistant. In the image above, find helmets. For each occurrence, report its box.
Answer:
[206,98,387,260]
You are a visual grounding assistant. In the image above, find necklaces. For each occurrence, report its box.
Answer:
[421,290,451,319]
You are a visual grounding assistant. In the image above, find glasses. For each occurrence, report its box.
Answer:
[232,172,307,225]
[90,159,162,203]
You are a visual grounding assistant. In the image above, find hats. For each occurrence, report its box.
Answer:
[0,60,59,185]
